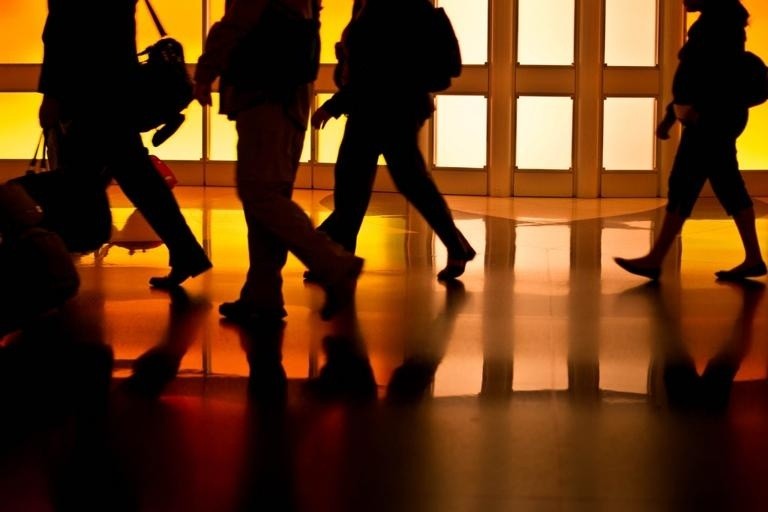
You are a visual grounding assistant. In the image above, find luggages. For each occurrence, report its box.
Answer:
[0,182,83,332]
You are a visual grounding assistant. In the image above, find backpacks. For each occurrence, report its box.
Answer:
[424,7,460,89]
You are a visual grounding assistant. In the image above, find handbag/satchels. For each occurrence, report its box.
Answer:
[743,48,767,108]
[5,165,110,252]
[136,39,190,134]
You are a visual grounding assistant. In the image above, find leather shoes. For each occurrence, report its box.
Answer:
[148,249,213,287]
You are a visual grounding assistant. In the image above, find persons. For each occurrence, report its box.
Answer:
[37,1,213,285]
[304,0,476,279]
[188,1,365,321]
[612,1,767,282]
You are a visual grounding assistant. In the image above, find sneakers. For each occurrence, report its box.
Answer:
[436,247,475,280]
[317,254,362,319]
[715,263,766,279]
[614,256,660,280]
[219,297,288,320]
[304,270,310,280]
